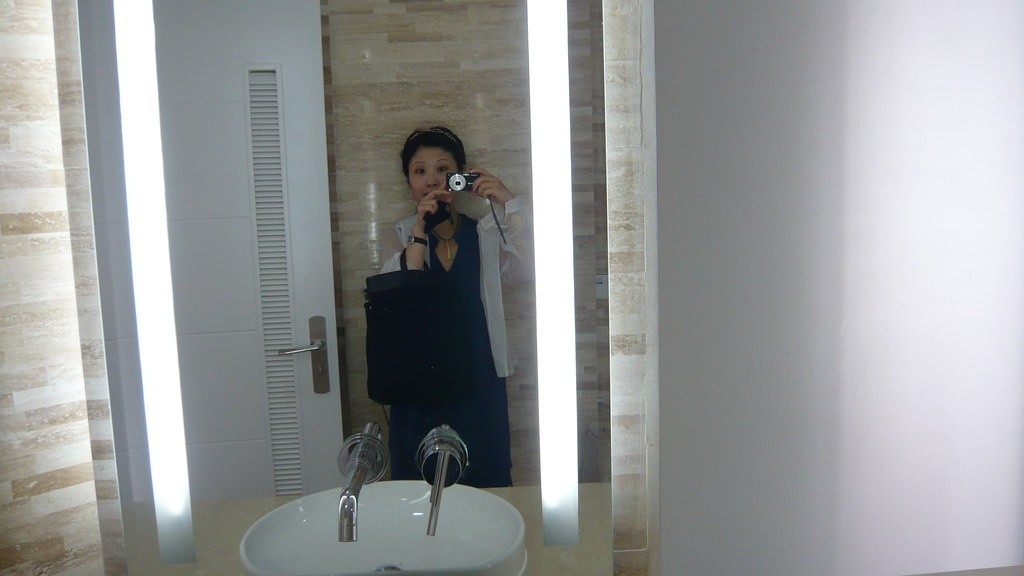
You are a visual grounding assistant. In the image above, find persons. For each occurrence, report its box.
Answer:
[377,126,527,488]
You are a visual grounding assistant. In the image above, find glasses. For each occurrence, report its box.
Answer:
[408,129,460,151]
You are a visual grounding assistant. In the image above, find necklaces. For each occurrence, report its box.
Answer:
[433,229,456,260]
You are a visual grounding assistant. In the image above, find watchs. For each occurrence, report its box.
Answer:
[407,235,428,246]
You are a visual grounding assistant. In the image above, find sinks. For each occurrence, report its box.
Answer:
[239,478,529,576]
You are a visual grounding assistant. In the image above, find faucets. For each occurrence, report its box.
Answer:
[362,420,382,440]
[336,461,370,543]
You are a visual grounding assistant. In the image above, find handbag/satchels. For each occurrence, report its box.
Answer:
[364,249,486,406]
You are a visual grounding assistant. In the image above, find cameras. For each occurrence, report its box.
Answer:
[446,173,480,191]
